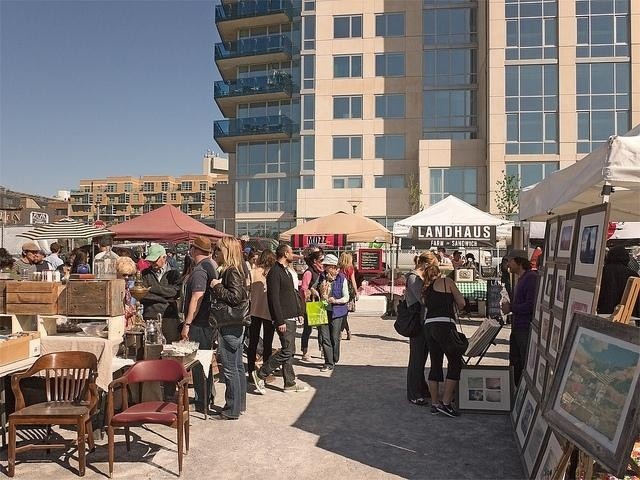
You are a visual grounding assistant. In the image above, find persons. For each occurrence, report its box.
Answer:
[1,236,140,326]
[597,246,640,326]
[404,243,541,416]
[137,235,362,420]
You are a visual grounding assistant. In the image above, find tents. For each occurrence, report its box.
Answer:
[391,194,514,300]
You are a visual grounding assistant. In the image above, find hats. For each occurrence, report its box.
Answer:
[453,251,462,256]
[192,236,212,252]
[321,254,338,265]
[21,244,39,251]
[145,244,166,262]
[504,249,528,259]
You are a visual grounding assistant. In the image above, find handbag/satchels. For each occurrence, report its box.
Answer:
[449,329,468,356]
[394,300,421,338]
[305,297,329,327]
[207,297,252,329]
[348,281,355,302]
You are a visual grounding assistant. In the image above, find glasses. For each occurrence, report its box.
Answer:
[122,274,132,277]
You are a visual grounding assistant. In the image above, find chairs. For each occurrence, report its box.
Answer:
[105,360,190,478]
[8,351,99,476]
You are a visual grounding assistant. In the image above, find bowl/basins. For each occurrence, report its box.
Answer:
[78,322,108,333]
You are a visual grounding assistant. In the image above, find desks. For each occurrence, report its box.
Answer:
[357,284,406,316]
[1,357,37,450]
[114,350,216,418]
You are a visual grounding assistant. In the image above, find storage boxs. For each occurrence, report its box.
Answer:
[0,277,126,365]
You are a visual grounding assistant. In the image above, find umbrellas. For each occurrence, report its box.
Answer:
[17,217,116,249]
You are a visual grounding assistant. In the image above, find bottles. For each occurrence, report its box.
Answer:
[33,269,60,283]
[501,296,508,304]
[149,323,157,344]
[21,269,29,281]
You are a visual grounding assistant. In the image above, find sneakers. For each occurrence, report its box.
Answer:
[302,353,310,360]
[252,370,266,394]
[409,399,427,405]
[321,366,333,372]
[211,410,245,420]
[284,382,308,393]
[347,332,351,339]
[432,400,456,418]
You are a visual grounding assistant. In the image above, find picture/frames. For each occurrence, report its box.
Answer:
[358,248,382,273]
